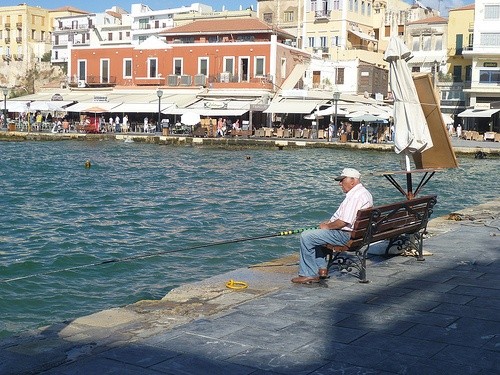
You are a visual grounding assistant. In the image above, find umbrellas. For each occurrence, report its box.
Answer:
[348,112,388,143]
[82,105,108,132]
[379,29,434,253]
[181,110,202,126]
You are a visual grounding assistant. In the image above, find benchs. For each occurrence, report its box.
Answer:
[230,129,252,138]
[317,193,439,283]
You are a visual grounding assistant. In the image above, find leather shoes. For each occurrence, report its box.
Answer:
[292,276,320,283]
[319,269,326,276]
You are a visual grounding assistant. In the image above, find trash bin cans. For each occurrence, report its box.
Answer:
[341,133,347,143]
[162,122,169,136]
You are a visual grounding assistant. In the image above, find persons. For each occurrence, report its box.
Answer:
[0,109,243,138]
[277,120,396,144]
[292,166,375,284]
[445,122,464,140]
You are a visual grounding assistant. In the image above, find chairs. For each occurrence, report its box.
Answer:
[254,127,277,138]
[302,129,308,139]
[318,130,324,139]
[284,128,292,138]
[295,129,302,139]
[277,129,284,138]
[462,131,500,142]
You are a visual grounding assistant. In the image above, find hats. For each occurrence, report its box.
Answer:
[335,168,361,181]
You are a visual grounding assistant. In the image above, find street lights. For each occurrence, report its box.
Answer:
[157,90,164,132]
[3,88,9,128]
[333,91,341,138]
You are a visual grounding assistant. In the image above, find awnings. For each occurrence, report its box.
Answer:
[262,98,396,120]
[457,108,500,118]
[0,92,259,118]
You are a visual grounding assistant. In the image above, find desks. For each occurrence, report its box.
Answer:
[262,127,274,132]
